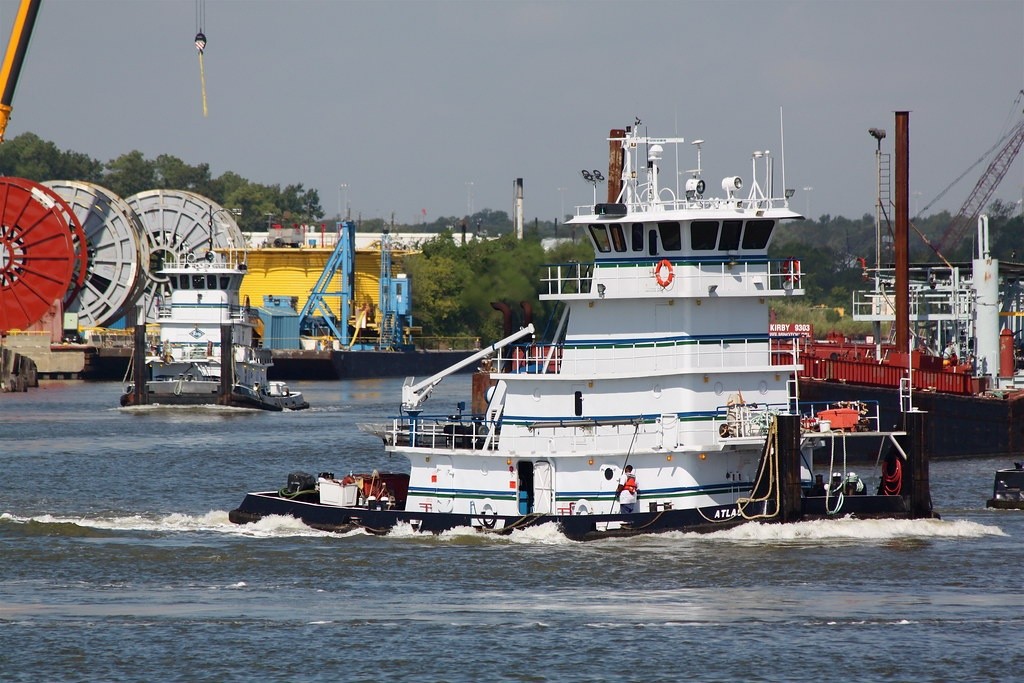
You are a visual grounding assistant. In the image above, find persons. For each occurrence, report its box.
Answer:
[614,464,639,527]
[942,341,957,368]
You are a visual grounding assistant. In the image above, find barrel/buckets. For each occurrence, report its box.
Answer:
[819,420,831,433]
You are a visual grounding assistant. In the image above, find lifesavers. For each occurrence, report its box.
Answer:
[783,255,801,284]
[655,260,674,288]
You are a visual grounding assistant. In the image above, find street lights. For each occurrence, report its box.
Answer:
[869,127,886,344]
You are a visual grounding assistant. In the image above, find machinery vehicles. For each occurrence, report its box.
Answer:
[0,0,208,141]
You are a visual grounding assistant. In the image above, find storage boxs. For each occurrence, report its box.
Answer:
[318,477,359,506]
[818,409,860,432]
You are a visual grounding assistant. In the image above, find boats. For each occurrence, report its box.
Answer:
[229,116,936,541]
[986,462,1024,511]
[119,205,310,412]
[490,214,1024,455]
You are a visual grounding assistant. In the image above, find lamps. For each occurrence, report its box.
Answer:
[786,189,795,197]
[722,176,742,197]
[686,179,705,197]
[597,284,605,292]
[708,284,719,294]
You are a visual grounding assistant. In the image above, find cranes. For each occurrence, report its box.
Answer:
[909,89,1024,262]
